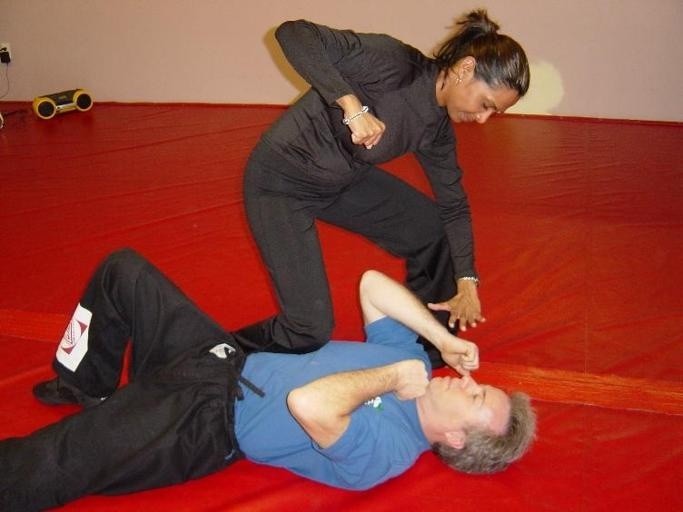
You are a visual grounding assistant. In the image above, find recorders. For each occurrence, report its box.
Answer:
[32,88,93,119]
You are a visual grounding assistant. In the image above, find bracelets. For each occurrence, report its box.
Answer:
[457,276,481,287]
[342,104,370,125]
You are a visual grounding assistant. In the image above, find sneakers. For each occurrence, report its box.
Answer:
[33,375,111,409]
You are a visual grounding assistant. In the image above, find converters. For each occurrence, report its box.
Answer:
[1,52,10,62]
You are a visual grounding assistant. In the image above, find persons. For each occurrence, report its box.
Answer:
[0,247,536,512]
[230,7,532,354]
